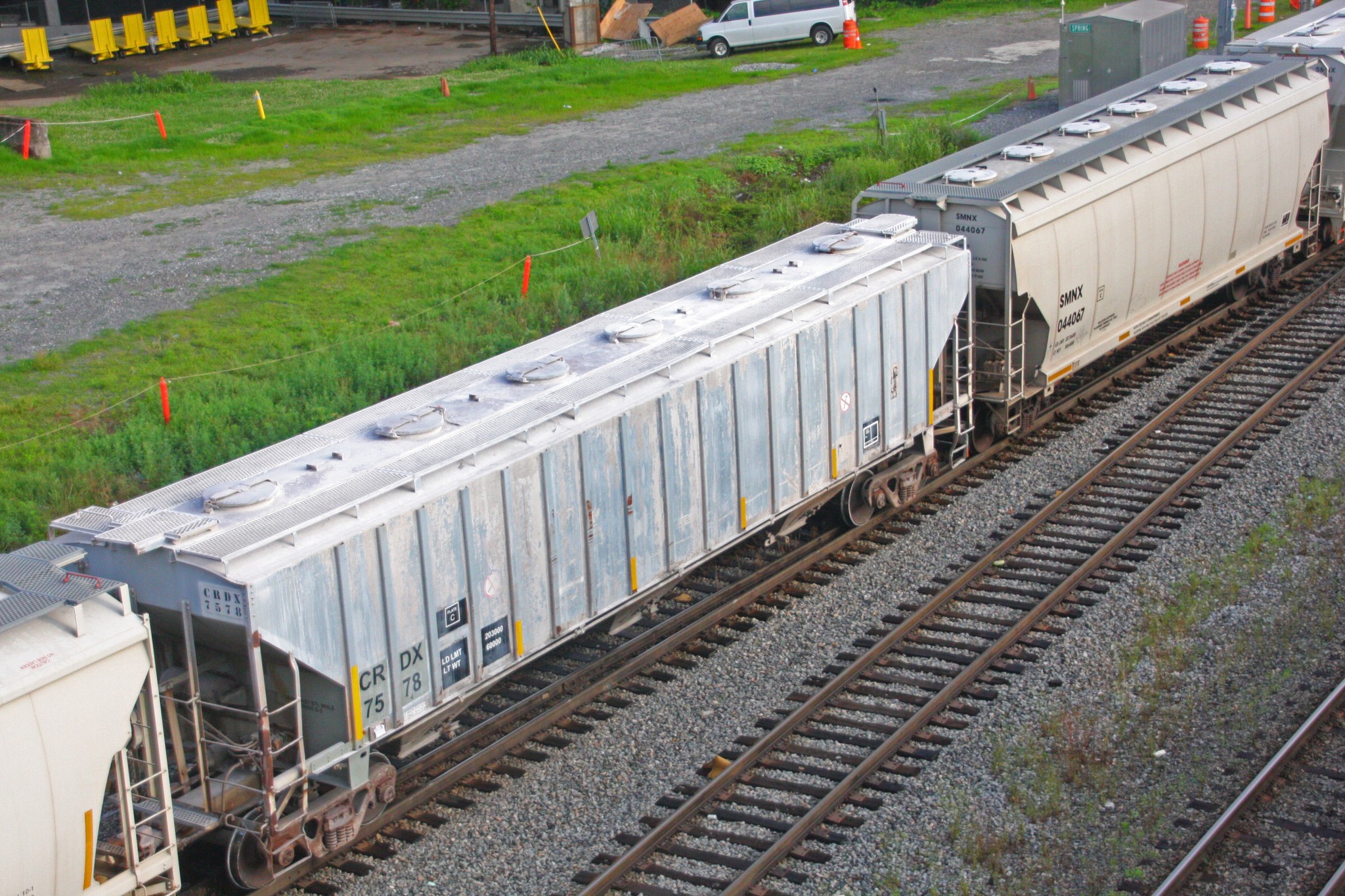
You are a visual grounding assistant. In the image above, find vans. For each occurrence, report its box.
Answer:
[696,0,859,58]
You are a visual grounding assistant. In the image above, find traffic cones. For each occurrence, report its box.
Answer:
[1027,75,1040,101]
[1239,0,1255,31]
[853,26,865,49]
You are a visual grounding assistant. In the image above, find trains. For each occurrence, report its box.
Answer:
[0,0,1345,896]
